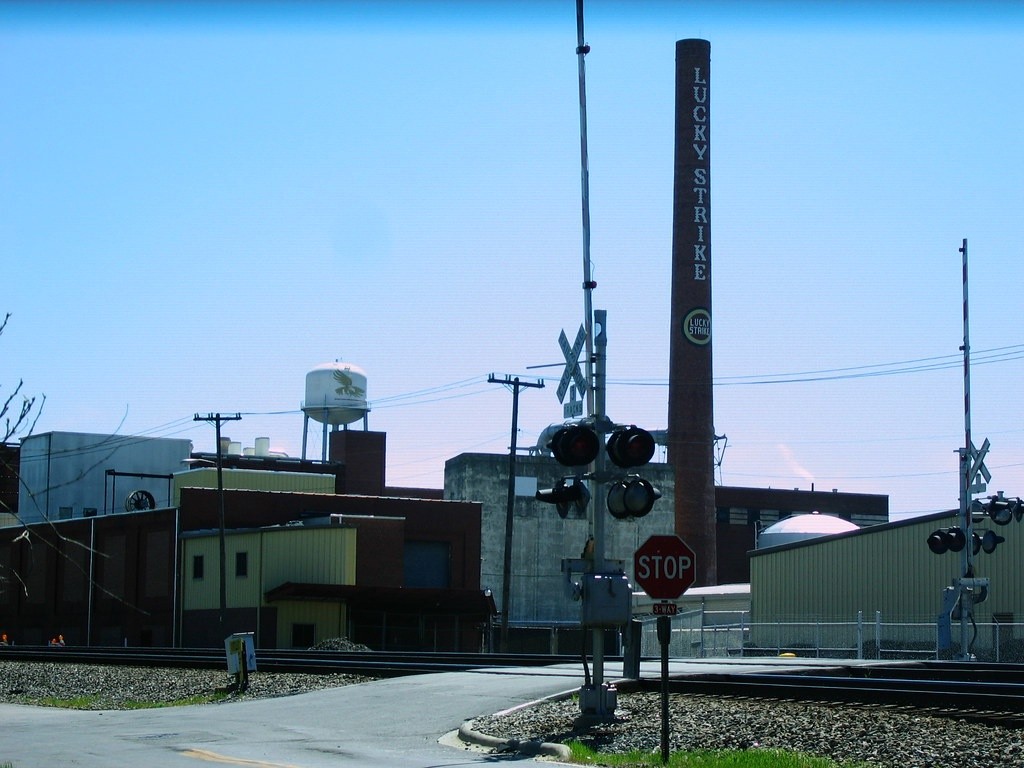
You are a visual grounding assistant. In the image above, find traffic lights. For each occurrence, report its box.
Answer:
[927,530,951,554]
[607,429,655,470]
[551,427,600,466]
[536,477,581,519]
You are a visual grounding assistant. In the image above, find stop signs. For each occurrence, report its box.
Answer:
[635,535,696,599]
